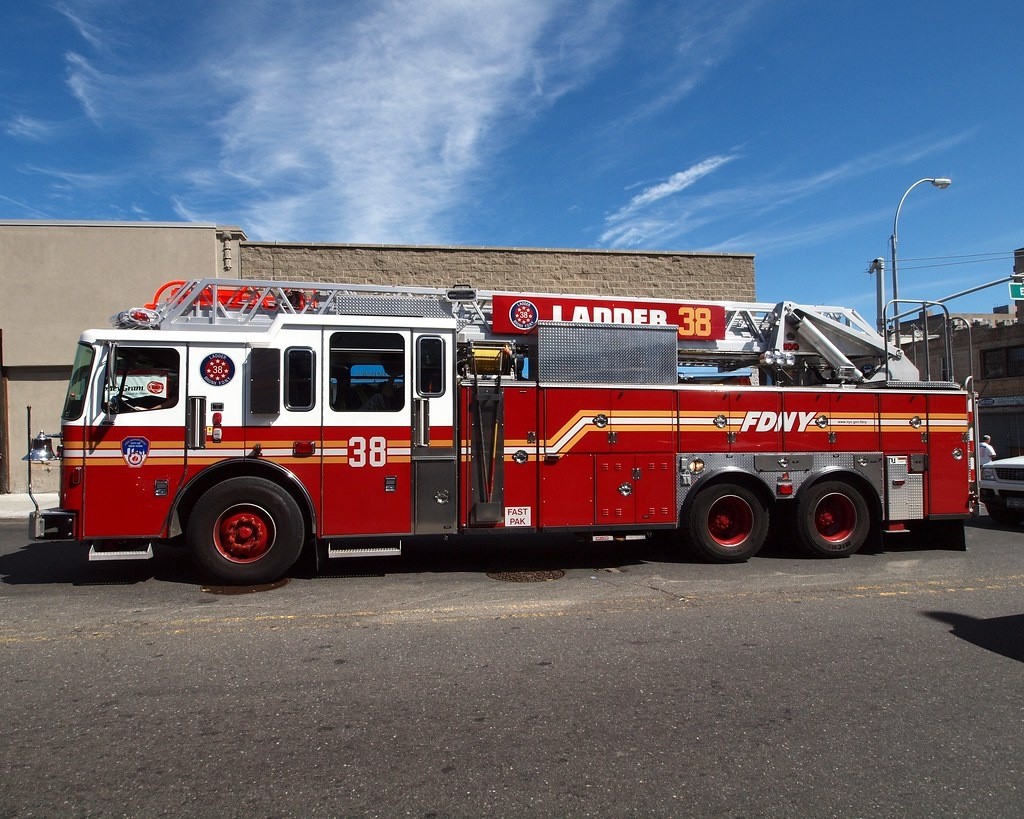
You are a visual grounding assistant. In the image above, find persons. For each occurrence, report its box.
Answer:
[131,397,178,412]
[980,435,996,469]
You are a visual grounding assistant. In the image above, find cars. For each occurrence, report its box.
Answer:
[980,457,1024,525]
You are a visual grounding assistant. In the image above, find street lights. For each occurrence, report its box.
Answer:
[891,177,952,348]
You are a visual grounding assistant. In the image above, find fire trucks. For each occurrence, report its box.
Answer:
[22,278,984,588]
